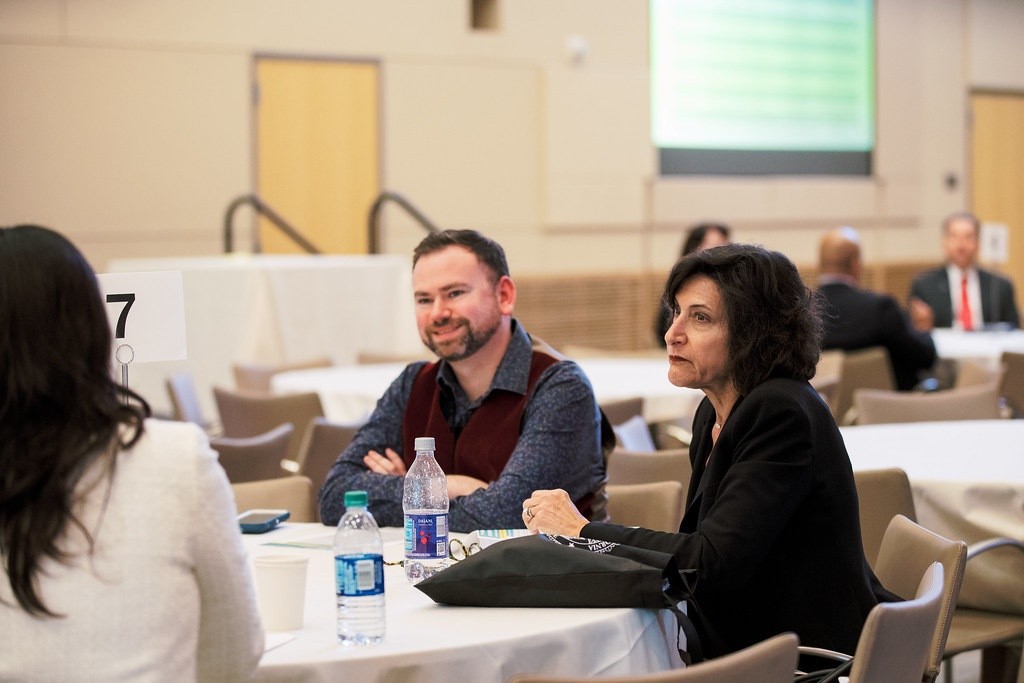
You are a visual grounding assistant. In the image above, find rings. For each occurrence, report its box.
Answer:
[527,506,533,518]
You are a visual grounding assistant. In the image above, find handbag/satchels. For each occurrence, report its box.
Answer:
[415,532,718,664]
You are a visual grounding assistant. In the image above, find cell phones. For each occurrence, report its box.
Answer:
[237,509,291,533]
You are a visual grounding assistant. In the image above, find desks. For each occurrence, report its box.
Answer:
[268,355,705,425]
[239,522,688,683]
[839,419,1024,615]
[931,326,1024,385]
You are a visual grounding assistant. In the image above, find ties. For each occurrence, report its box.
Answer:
[960,275,973,334]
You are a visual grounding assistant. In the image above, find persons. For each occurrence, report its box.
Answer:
[317,230,616,533]
[521,246,908,683]
[654,223,732,348]
[0,225,265,683]
[808,229,936,392]
[910,214,1021,331]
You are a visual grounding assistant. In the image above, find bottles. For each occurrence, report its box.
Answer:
[333,491,387,644]
[403,437,450,587]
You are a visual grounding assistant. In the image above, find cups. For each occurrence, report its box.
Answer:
[252,553,310,632]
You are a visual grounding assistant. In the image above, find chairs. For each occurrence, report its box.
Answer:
[161,347,1024,683]
